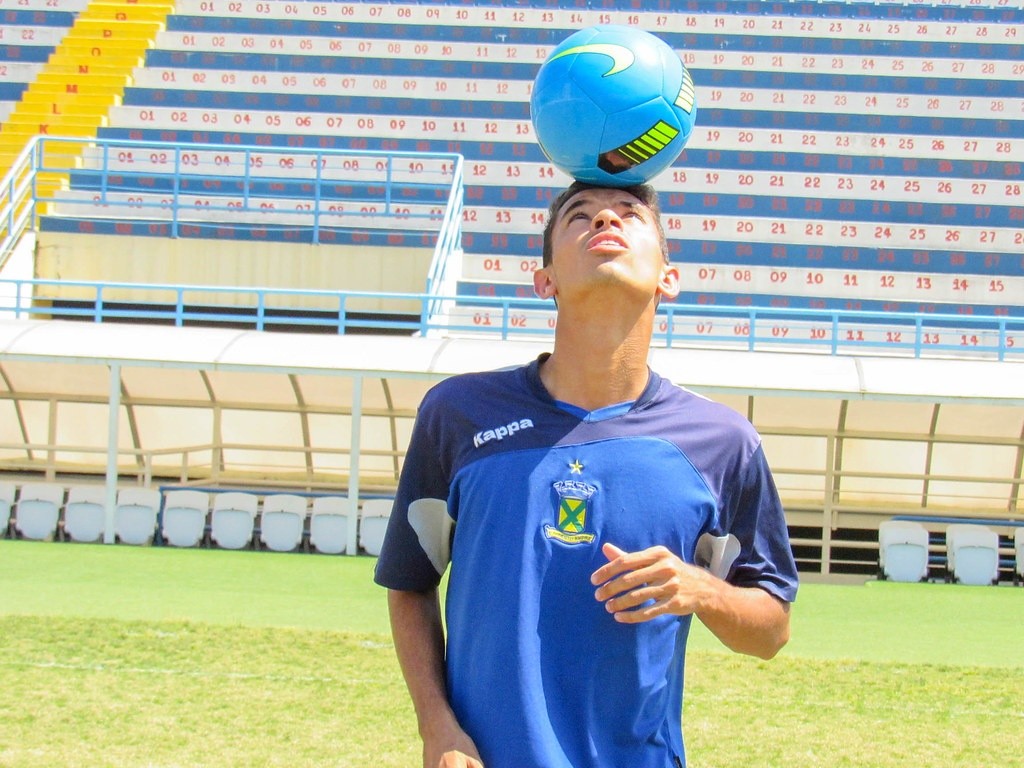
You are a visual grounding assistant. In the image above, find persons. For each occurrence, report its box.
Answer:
[373,181,799,768]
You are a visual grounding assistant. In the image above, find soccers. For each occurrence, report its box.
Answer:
[532,23,699,190]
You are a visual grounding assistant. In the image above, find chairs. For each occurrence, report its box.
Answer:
[0,480,17,536]
[946,524,999,585]
[211,492,259,550]
[16,482,64,540]
[260,494,308,552]
[113,487,162,545]
[162,490,209,547]
[45,0,1024,362]
[65,485,107,542]
[310,496,352,554]
[1015,527,1024,585]
[0,0,86,123]
[359,498,395,556]
[875,520,929,582]
